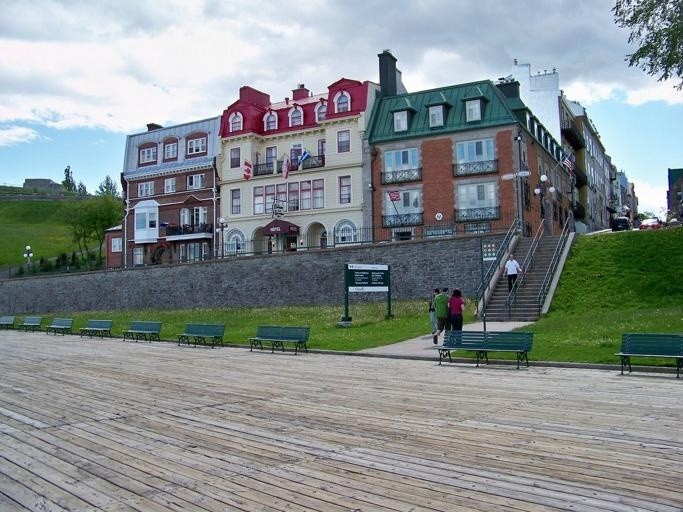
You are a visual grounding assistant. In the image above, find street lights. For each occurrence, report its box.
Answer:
[216,218,229,259]
[24,246,33,277]
[535,174,555,218]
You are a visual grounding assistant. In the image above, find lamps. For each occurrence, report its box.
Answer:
[368,183,376,191]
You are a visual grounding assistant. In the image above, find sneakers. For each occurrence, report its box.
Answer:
[434,335,437,344]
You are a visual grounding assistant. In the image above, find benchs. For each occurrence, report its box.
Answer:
[80,319,113,339]
[176,323,226,348]
[434,330,533,369]
[123,321,163,342]
[614,334,683,378]
[18,317,43,333]
[0,316,16,330]
[45,318,74,336]
[248,326,310,355]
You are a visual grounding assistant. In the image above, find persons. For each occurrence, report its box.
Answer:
[503,253,522,293]
[448,289,464,344]
[429,289,440,335]
[433,288,450,344]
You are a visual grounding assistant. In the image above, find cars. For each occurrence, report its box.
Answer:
[612,217,633,231]
[639,218,660,229]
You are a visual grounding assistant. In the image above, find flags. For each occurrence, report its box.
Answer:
[298,150,311,166]
[564,151,576,170]
[244,161,251,180]
[388,191,401,201]
[282,155,291,179]
